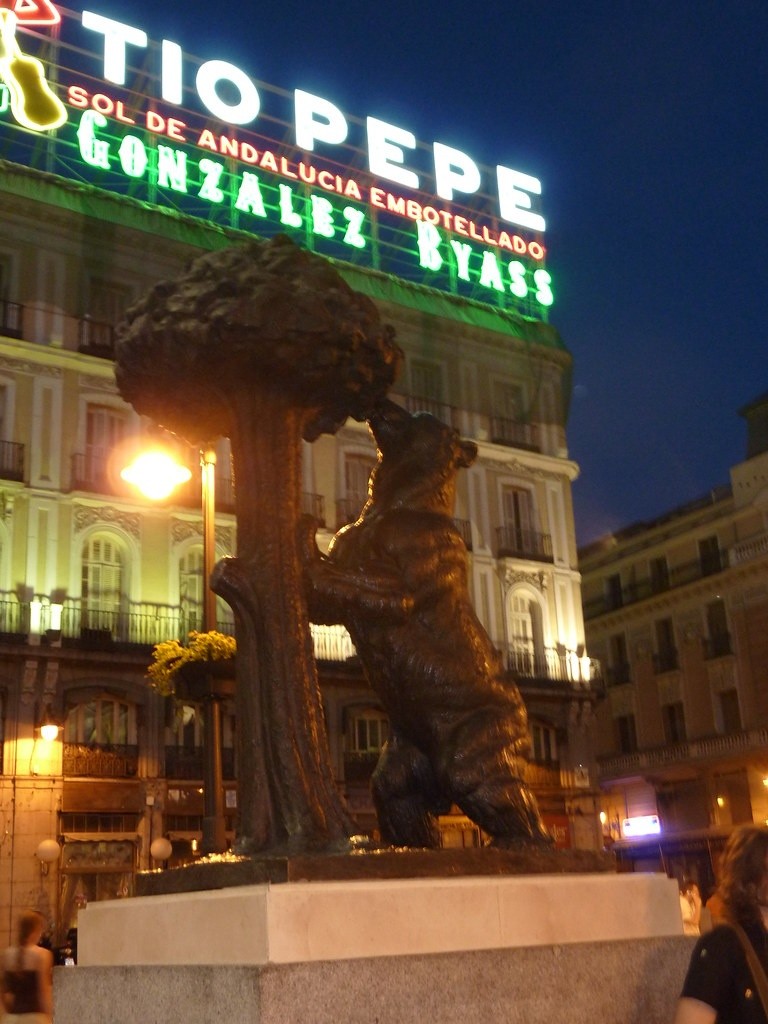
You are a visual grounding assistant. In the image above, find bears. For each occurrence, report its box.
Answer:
[295,398,559,852]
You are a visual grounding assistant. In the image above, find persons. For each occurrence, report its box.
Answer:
[677,878,705,934]
[0,910,56,1024]
[674,822,767,1024]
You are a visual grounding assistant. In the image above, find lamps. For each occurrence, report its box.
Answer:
[35,838,61,877]
[149,832,174,860]
[34,701,65,743]
